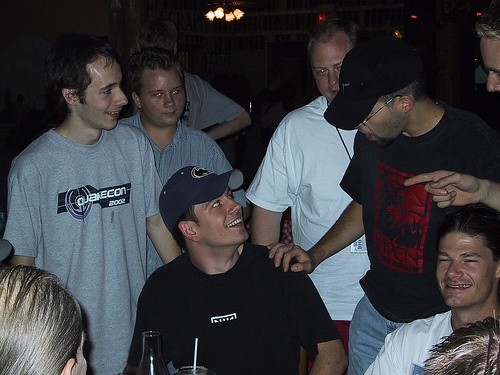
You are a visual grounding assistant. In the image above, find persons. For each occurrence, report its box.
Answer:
[242,16,372,375]
[2,33,184,375]
[267,35,500,375]
[0,167,500,375]
[118,47,251,281]
[139,19,252,140]
[404,0,500,212]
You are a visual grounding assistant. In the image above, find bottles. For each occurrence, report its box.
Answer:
[136,331,170,375]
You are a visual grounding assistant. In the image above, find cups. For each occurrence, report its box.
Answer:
[175,366,207,375]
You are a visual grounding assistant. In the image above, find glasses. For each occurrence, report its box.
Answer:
[352,94,403,129]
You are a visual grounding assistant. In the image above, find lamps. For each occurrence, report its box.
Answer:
[205,0,244,22]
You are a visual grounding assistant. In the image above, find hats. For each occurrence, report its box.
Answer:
[158,165,245,233]
[324,40,422,130]
